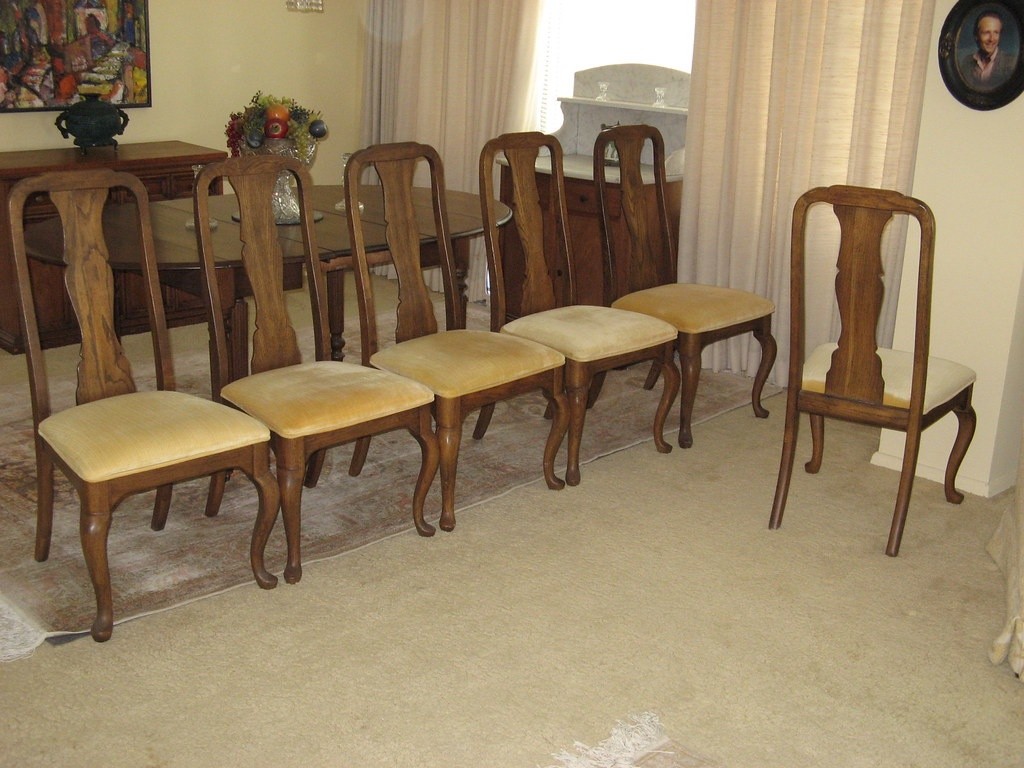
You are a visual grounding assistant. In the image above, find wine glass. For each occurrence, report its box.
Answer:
[185,164,219,229]
[335,152,365,212]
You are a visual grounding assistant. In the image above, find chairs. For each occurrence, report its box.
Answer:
[344,141,570,533]
[193,154,442,587]
[473,132,682,488]
[769,184,976,557]
[7,171,280,644]
[586,124,777,448]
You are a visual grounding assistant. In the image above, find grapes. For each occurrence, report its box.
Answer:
[225,94,311,159]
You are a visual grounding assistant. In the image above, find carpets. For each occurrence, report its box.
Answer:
[0,294,782,659]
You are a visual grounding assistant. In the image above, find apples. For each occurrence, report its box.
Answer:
[267,104,288,122]
[265,118,287,139]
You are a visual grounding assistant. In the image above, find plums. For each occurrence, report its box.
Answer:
[246,129,263,148]
[309,120,326,137]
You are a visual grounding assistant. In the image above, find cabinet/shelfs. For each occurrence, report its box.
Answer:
[498,165,681,308]
[0,139,229,358]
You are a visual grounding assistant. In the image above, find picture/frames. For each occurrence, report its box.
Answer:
[938,0,1024,111]
[0,1,152,113]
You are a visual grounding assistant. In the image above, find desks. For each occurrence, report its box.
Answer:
[24,185,511,370]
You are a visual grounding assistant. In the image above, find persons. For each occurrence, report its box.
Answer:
[961,10,1017,92]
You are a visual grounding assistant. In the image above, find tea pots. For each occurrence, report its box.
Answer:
[600,121,619,167]
